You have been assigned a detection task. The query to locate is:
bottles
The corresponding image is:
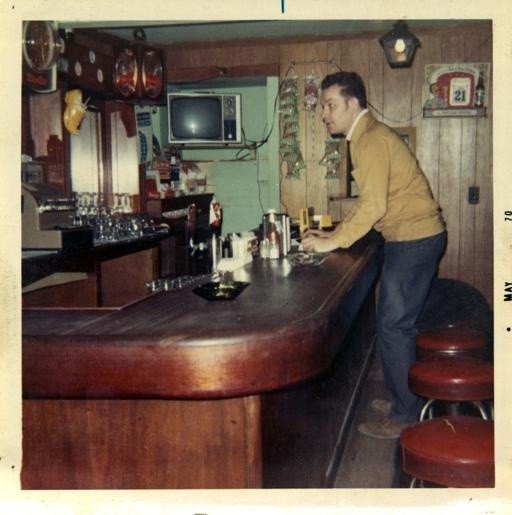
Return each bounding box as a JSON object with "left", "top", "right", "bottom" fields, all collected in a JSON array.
[
  {"left": 258, "top": 238, "right": 269, "bottom": 261},
  {"left": 474, "top": 70, "right": 485, "bottom": 108},
  {"left": 268, "top": 206, "right": 282, "bottom": 261}
]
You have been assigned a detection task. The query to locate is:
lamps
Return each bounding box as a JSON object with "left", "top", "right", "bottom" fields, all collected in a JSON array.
[{"left": 378, "top": 19, "right": 423, "bottom": 68}]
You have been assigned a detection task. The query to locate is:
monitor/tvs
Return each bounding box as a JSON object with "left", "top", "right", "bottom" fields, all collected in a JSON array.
[{"left": 167, "top": 93, "right": 243, "bottom": 143}]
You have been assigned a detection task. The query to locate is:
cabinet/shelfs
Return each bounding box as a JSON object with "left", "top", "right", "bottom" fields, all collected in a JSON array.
[{"left": 23, "top": 246, "right": 160, "bottom": 312}]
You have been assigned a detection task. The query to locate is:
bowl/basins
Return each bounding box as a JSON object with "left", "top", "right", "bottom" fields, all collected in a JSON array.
[{"left": 191, "top": 281, "right": 252, "bottom": 302}]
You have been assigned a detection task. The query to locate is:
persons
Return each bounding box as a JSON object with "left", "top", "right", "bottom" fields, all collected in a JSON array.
[
  {"left": 302, "top": 72, "right": 447, "bottom": 438},
  {"left": 424, "top": 83, "right": 448, "bottom": 108}
]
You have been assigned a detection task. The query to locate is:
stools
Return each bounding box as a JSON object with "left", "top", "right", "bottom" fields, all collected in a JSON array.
[{"left": 398, "top": 322, "right": 494, "bottom": 487}]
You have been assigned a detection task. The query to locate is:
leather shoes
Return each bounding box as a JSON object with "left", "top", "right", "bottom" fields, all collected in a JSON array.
[{"left": 356, "top": 398, "right": 401, "bottom": 440}]
